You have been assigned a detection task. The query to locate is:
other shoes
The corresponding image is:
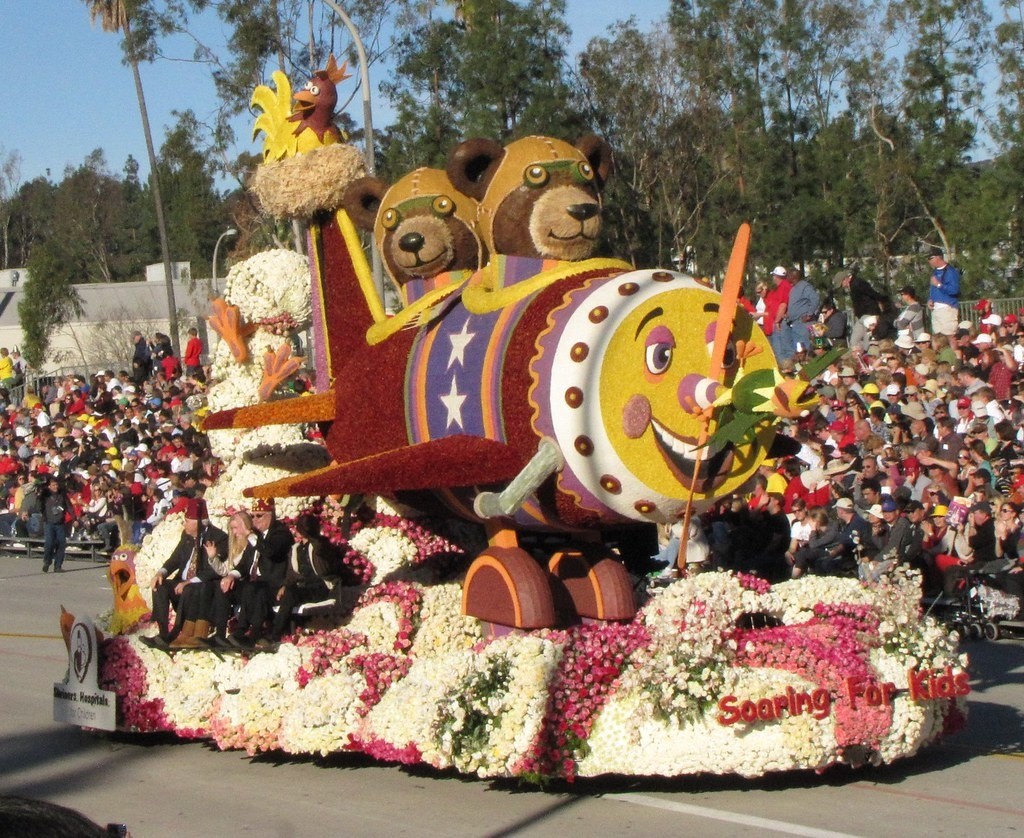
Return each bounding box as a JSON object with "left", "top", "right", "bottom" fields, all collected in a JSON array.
[
  {"left": 54, "top": 568, "right": 64, "bottom": 573},
  {"left": 42, "top": 563, "right": 49, "bottom": 572}
]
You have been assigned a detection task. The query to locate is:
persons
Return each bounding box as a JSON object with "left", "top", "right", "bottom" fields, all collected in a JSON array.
[
  {"left": 170, "top": 511, "right": 256, "bottom": 647},
  {"left": 230, "top": 513, "right": 342, "bottom": 655},
  {"left": 2, "top": 240, "right": 1024, "bottom": 658},
  {"left": 137, "top": 497, "right": 229, "bottom": 658},
  {"left": 194, "top": 497, "right": 296, "bottom": 653}
]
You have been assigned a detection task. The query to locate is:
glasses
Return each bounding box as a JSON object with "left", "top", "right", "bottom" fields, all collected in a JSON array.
[{"left": 249, "top": 512, "right": 267, "bottom": 519}]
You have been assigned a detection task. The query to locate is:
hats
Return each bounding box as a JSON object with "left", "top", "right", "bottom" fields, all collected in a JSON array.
[
  {"left": 762, "top": 246, "right": 1023, "bottom": 523},
  {"left": 76, "top": 414, "right": 89, "bottom": 421},
  {"left": 104, "top": 446, "right": 118, "bottom": 455},
  {"left": 184, "top": 499, "right": 208, "bottom": 519},
  {"left": 251, "top": 496, "right": 276, "bottom": 512},
  {"left": 5, "top": 404, "right": 16, "bottom": 410},
  {"left": 33, "top": 403, "right": 43, "bottom": 408},
  {"left": 147, "top": 397, "right": 161, "bottom": 405},
  {"left": 53, "top": 427, "right": 68, "bottom": 437},
  {"left": 101, "top": 460, "right": 110, "bottom": 464},
  {"left": 134, "top": 443, "right": 148, "bottom": 451},
  {"left": 95, "top": 370, "right": 105, "bottom": 377}
]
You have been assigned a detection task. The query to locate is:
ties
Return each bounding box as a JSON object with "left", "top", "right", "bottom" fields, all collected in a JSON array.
[
  {"left": 187, "top": 538, "right": 198, "bottom": 581},
  {"left": 301, "top": 545, "right": 307, "bottom": 577},
  {"left": 250, "top": 533, "right": 263, "bottom": 583}
]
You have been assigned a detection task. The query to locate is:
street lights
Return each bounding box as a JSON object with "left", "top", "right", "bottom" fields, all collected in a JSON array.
[{"left": 211, "top": 228, "right": 238, "bottom": 294}]
